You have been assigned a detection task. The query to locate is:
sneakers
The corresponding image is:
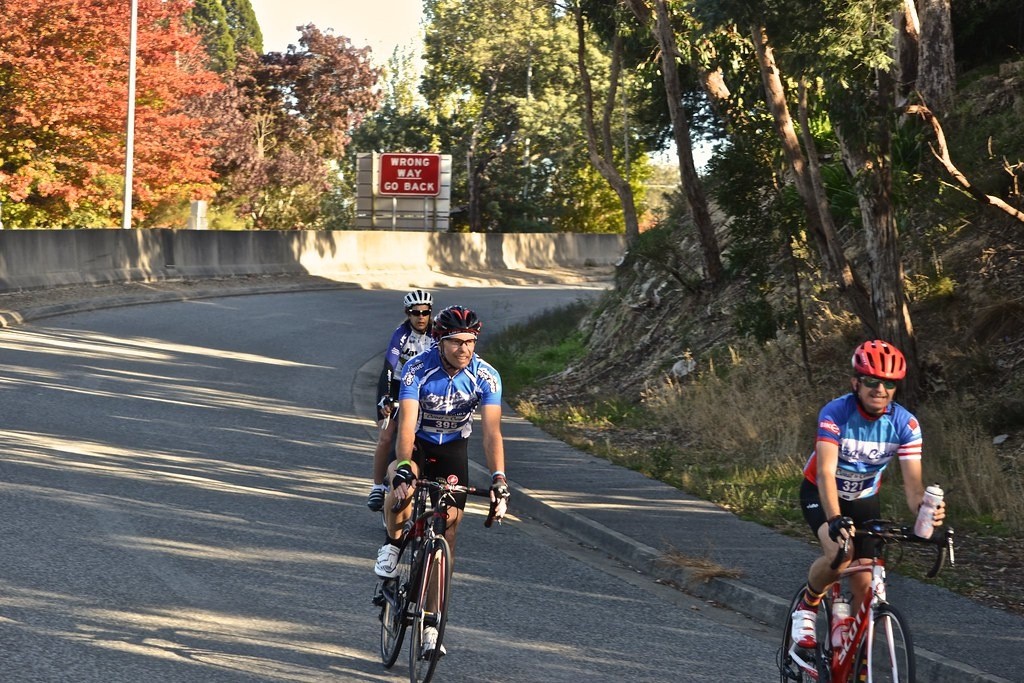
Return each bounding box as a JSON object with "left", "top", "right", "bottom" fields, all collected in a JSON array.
[
  {"left": 792, "top": 602, "right": 819, "bottom": 649},
  {"left": 374, "top": 544, "right": 401, "bottom": 579},
  {"left": 368, "top": 486, "right": 384, "bottom": 512},
  {"left": 421, "top": 627, "right": 446, "bottom": 661}
]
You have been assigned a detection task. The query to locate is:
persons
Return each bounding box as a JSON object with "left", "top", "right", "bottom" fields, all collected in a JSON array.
[
  {"left": 367, "top": 290, "right": 437, "bottom": 509},
  {"left": 374, "top": 305, "right": 509, "bottom": 662},
  {"left": 789, "top": 341, "right": 949, "bottom": 683}
]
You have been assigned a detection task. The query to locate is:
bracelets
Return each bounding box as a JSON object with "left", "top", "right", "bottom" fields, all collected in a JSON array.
[
  {"left": 492, "top": 472, "right": 506, "bottom": 480},
  {"left": 396, "top": 461, "right": 411, "bottom": 468}
]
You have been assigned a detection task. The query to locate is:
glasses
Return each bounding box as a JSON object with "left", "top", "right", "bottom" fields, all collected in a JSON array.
[
  {"left": 442, "top": 336, "right": 479, "bottom": 346},
  {"left": 409, "top": 309, "right": 432, "bottom": 316},
  {"left": 858, "top": 374, "right": 897, "bottom": 389}
]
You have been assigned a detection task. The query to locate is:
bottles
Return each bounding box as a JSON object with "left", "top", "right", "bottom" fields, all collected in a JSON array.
[
  {"left": 832, "top": 596, "right": 851, "bottom": 648},
  {"left": 915, "top": 482, "right": 944, "bottom": 539}
]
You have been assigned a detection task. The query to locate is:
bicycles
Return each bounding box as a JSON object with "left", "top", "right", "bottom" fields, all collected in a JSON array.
[
  {"left": 373, "top": 396, "right": 429, "bottom": 578},
  {"left": 774, "top": 516, "right": 956, "bottom": 683},
  {"left": 369, "top": 474, "right": 512, "bottom": 683}
]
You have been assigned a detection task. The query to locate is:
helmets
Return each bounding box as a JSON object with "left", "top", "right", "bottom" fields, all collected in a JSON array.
[
  {"left": 404, "top": 290, "right": 433, "bottom": 309},
  {"left": 432, "top": 305, "right": 483, "bottom": 344},
  {"left": 851, "top": 340, "right": 907, "bottom": 380}
]
